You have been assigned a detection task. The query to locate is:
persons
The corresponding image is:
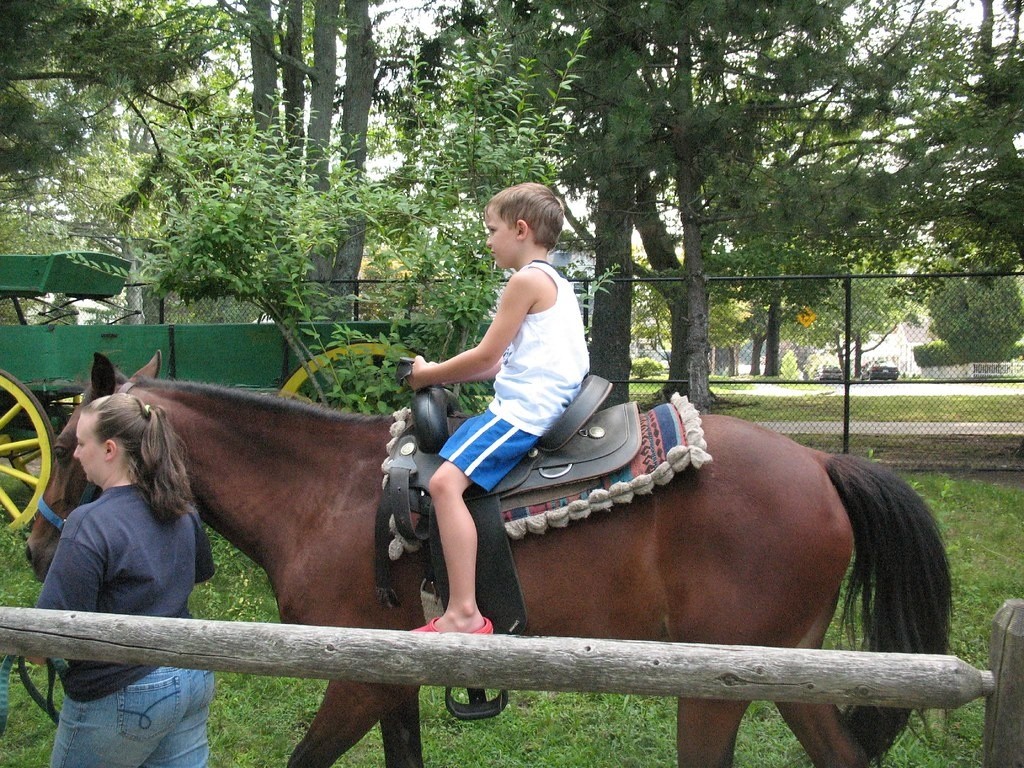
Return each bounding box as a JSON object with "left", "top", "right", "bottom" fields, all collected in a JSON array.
[
  {"left": 27, "top": 395, "right": 214, "bottom": 768},
  {"left": 405, "top": 182, "right": 592, "bottom": 633}
]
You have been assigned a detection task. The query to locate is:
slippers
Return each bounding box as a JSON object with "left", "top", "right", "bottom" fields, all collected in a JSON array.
[{"left": 412, "top": 618, "right": 493, "bottom": 634}]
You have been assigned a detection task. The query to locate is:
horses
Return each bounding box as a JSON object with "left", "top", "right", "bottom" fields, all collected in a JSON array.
[{"left": 24, "top": 351, "right": 950, "bottom": 768}]
